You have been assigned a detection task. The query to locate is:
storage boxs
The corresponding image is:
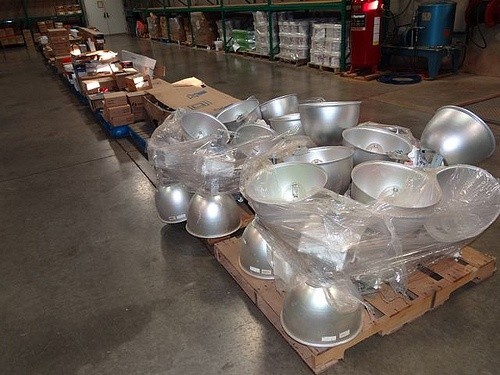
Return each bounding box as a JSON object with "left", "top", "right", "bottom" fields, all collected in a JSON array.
[{"left": 0, "top": 5, "right": 248, "bottom": 127}]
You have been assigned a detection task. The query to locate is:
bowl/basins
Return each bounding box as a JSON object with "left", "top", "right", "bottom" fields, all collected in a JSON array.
[
  {"left": 245, "top": 162, "right": 327, "bottom": 221},
  {"left": 419, "top": 105, "right": 495, "bottom": 166},
  {"left": 424, "top": 163, "right": 500, "bottom": 243},
  {"left": 340, "top": 125, "right": 412, "bottom": 162},
  {"left": 238, "top": 220, "right": 285, "bottom": 280},
  {"left": 187, "top": 190, "right": 243, "bottom": 241},
  {"left": 280, "top": 275, "right": 364, "bottom": 347},
  {"left": 215, "top": 99, "right": 261, "bottom": 131},
  {"left": 232, "top": 123, "right": 275, "bottom": 146},
  {"left": 258, "top": 92, "right": 302, "bottom": 120},
  {"left": 154, "top": 177, "right": 188, "bottom": 223},
  {"left": 279, "top": 146, "right": 354, "bottom": 197},
  {"left": 269, "top": 113, "right": 302, "bottom": 136},
  {"left": 299, "top": 99, "right": 363, "bottom": 145},
  {"left": 347, "top": 159, "right": 441, "bottom": 236},
  {"left": 178, "top": 113, "right": 229, "bottom": 150}
]
